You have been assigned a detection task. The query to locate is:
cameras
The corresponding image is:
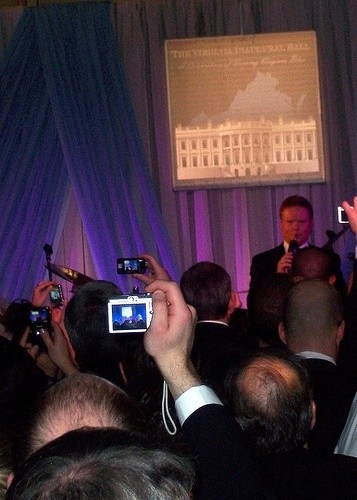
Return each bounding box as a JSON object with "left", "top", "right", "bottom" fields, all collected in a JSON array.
[
  {"left": 116, "top": 258, "right": 147, "bottom": 275},
  {"left": 106, "top": 293, "right": 153, "bottom": 334},
  {"left": 49, "top": 284, "right": 64, "bottom": 308},
  {"left": 337, "top": 206, "right": 354, "bottom": 224},
  {"left": 29, "top": 306, "right": 51, "bottom": 335}
]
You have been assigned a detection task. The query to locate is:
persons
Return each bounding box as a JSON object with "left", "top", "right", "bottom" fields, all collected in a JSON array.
[
  {"left": 8, "top": 280, "right": 257, "bottom": 500},
  {"left": 0, "top": 196, "right": 357, "bottom": 500},
  {"left": 247, "top": 195, "right": 349, "bottom": 331}
]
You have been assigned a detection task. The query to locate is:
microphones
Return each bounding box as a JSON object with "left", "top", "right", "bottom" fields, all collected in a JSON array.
[{"left": 287, "top": 239, "right": 298, "bottom": 273}]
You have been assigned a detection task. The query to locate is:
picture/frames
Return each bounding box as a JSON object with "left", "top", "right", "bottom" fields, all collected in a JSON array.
[{"left": 161, "top": 31, "right": 328, "bottom": 192}]
